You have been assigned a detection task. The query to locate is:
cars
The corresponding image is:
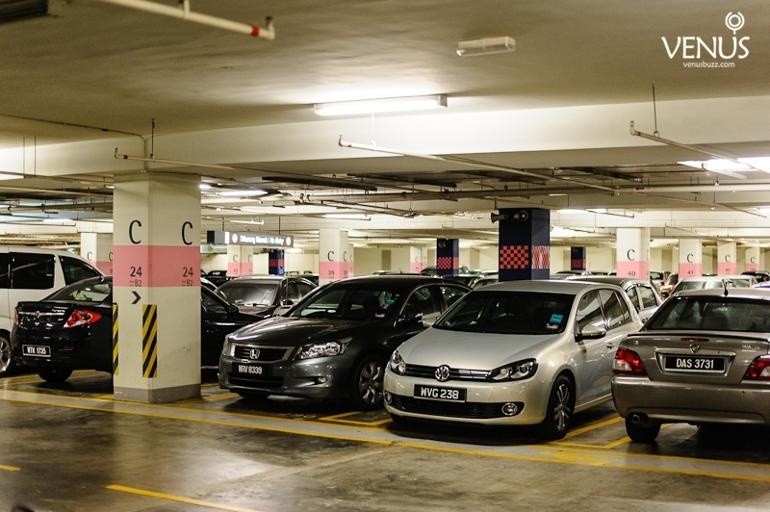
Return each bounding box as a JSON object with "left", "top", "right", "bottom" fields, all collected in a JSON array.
[{"left": 1, "top": 241, "right": 769, "bottom": 457}]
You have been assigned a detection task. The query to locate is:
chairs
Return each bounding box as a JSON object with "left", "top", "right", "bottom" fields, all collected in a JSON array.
[
  {"left": 362, "top": 296, "right": 380, "bottom": 317},
  {"left": 701, "top": 311, "right": 731, "bottom": 330}
]
